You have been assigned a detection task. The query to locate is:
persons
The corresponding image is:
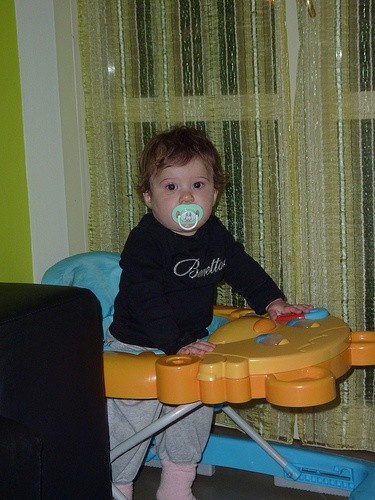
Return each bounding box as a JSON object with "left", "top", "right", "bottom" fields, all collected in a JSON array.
[{"left": 100, "top": 124, "right": 314, "bottom": 499}]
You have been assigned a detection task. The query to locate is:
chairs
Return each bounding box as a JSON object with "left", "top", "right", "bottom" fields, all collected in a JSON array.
[{"left": 38, "top": 253, "right": 226, "bottom": 415}]
